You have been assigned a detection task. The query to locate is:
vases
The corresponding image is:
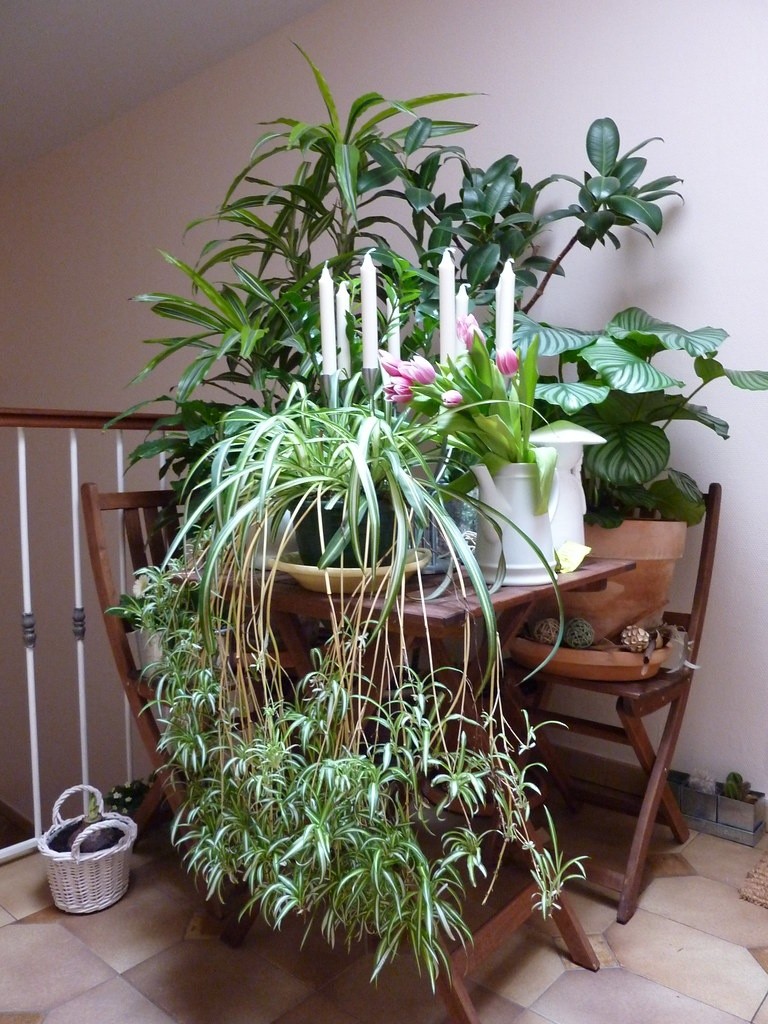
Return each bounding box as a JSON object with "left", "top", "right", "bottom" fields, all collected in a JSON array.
[{"left": 475, "top": 459, "right": 559, "bottom": 587}]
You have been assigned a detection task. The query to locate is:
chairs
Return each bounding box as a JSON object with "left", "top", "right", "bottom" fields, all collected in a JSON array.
[
  {"left": 82, "top": 480, "right": 350, "bottom": 920},
  {"left": 489, "top": 480, "right": 724, "bottom": 925}
]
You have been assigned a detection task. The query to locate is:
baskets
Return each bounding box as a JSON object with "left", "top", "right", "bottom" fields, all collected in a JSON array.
[{"left": 38, "top": 784, "right": 138, "bottom": 915}]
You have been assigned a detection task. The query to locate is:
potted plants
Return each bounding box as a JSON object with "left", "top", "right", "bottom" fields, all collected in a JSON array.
[{"left": 100, "top": 84, "right": 710, "bottom": 989}]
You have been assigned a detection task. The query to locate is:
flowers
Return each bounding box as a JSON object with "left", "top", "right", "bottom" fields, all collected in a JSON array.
[
  {"left": 379, "top": 311, "right": 544, "bottom": 464},
  {"left": 114, "top": 557, "right": 160, "bottom": 624}
]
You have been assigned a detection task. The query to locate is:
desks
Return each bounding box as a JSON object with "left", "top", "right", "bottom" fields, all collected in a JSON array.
[{"left": 168, "top": 558, "right": 639, "bottom": 1024}]
